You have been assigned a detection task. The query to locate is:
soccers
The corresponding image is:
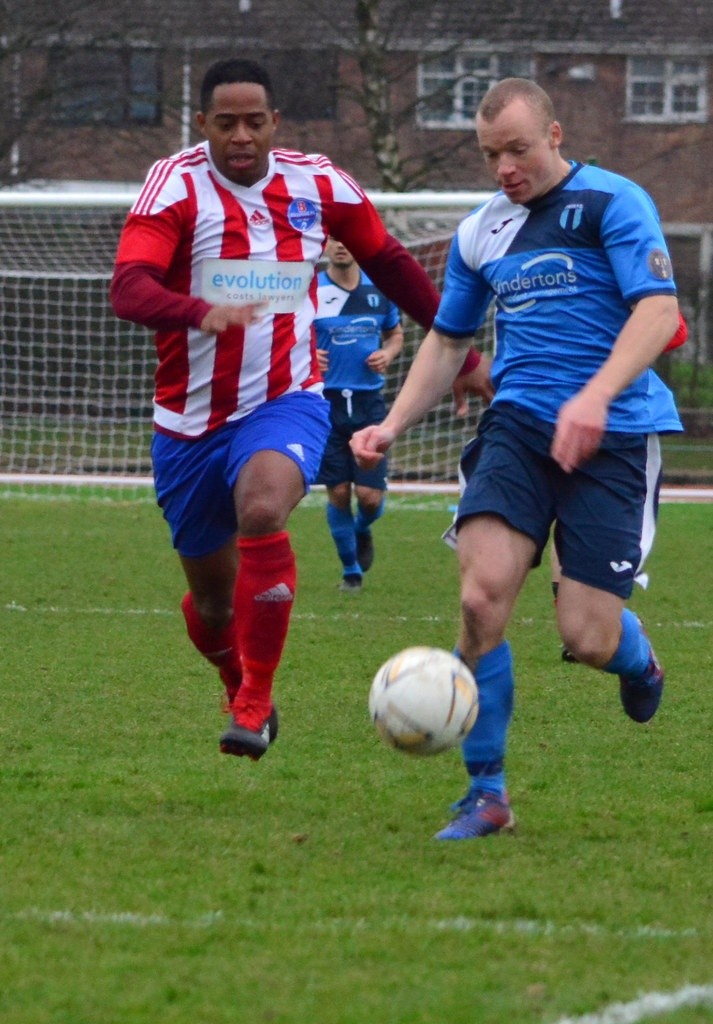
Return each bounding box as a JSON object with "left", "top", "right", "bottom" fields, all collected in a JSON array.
[{"left": 367, "top": 645, "right": 480, "bottom": 756}]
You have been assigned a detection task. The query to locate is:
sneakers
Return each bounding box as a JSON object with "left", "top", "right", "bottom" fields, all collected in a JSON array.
[
  {"left": 355, "top": 529, "right": 373, "bottom": 572},
  {"left": 219, "top": 707, "right": 278, "bottom": 760},
  {"left": 619, "top": 616, "right": 664, "bottom": 722},
  {"left": 430, "top": 786, "right": 518, "bottom": 839},
  {"left": 340, "top": 574, "right": 361, "bottom": 591}
]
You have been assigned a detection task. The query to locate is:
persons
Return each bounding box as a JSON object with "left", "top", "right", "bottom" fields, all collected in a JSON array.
[
  {"left": 346, "top": 78, "right": 688, "bottom": 847},
  {"left": 315, "top": 232, "right": 406, "bottom": 594},
  {"left": 108, "top": 59, "right": 497, "bottom": 761},
  {"left": 552, "top": 307, "right": 688, "bottom": 664}
]
